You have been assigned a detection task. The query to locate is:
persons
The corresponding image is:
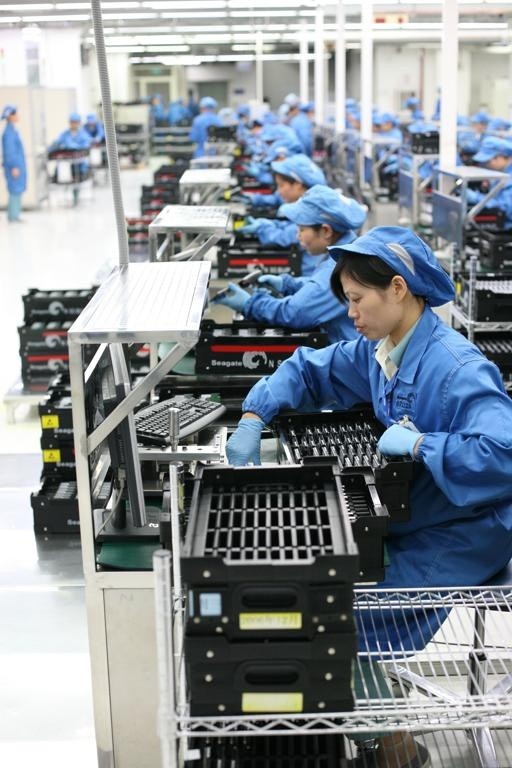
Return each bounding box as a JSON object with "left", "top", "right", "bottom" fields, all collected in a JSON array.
[
  {"left": 0, "top": 104, "right": 30, "bottom": 222},
  {"left": 47, "top": 79, "right": 511, "bottom": 344},
  {"left": 222, "top": 223, "right": 511, "bottom": 767}
]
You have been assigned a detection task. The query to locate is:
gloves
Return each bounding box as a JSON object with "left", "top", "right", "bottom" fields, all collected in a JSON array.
[
  {"left": 226, "top": 420, "right": 263, "bottom": 466},
  {"left": 242, "top": 163, "right": 272, "bottom": 237},
  {"left": 378, "top": 423, "right": 428, "bottom": 461},
  {"left": 260, "top": 273, "right": 284, "bottom": 294},
  {"left": 216, "top": 283, "right": 250, "bottom": 315}
]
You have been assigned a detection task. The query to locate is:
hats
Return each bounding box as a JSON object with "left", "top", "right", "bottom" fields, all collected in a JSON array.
[
  {"left": 346, "top": 93, "right": 511, "bottom": 161},
  {"left": 69, "top": 115, "right": 97, "bottom": 127},
  {"left": 269, "top": 153, "right": 325, "bottom": 188},
  {"left": 0, "top": 106, "right": 16, "bottom": 120},
  {"left": 327, "top": 225, "right": 456, "bottom": 306},
  {"left": 152, "top": 92, "right": 316, "bottom": 162},
  {"left": 276, "top": 185, "right": 369, "bottom": 232}
]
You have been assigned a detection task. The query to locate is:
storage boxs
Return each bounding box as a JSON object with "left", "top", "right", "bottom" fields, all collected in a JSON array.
[{"left": 17, "top": 286, "right": 113, "bottom": 539}]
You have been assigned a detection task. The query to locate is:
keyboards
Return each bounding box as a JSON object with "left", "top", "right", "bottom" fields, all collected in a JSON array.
[{"left": 133, "top": 393, "right": 227, "bottom": 448}]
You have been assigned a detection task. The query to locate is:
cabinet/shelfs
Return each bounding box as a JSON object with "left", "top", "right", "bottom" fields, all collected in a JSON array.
[{"left": 44, "top": 151, "right": 96, "bottom": 208}]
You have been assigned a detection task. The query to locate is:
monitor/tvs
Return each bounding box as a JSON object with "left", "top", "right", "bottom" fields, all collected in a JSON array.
[{"left": 94, "top": 341, "right": 162, "bottom": 543}]
